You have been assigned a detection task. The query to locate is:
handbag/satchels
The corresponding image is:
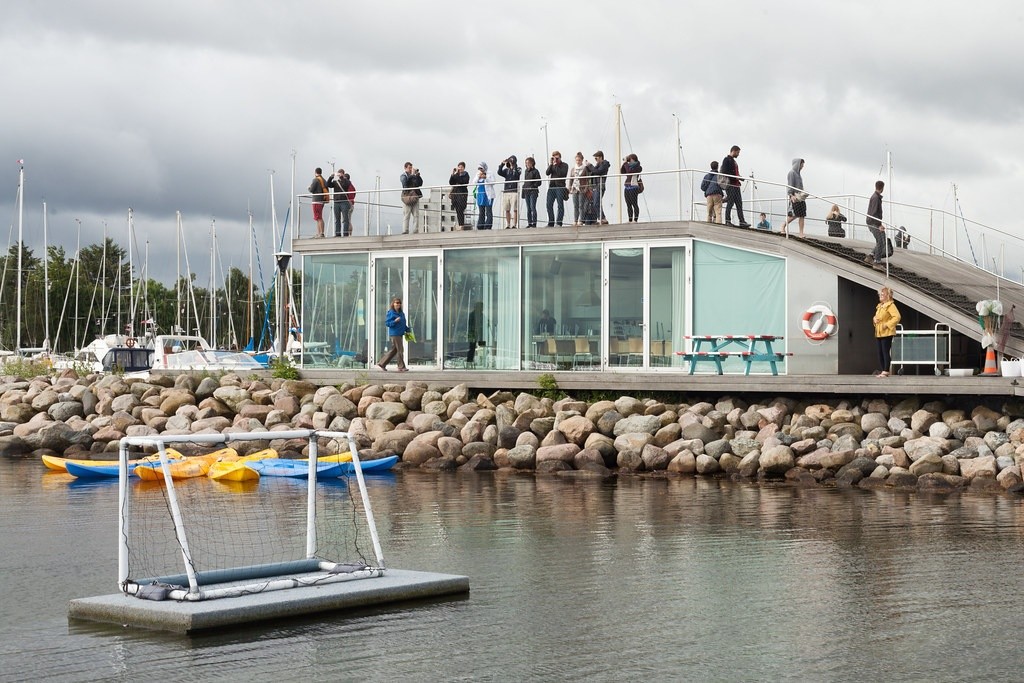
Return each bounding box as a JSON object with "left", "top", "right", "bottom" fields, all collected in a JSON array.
[
  {"left": 586, "top": 189, "right": 593, "bottom": 199},
  {"left": 449, "top": 191, "right": 453, "bottom": 199},
  {"left": 348, "top": 205, "right": 354, "bottom": 213},
  {"left": 881, "top": 238, "right": 893, "bottom": 258},
  {"left": 841, "top": 229, "right": 844, "bottom": 237},
  {"left": 564, "top": 189, "right": 569, "bottom": 201},
  {"left": 522, "top": 186, "right": 525, "bottom": 198},
  {"left": 637, "top": 179, "right": 644, "bottom": 194},
  {"left": 472, "top": 186, "right": 477, "bottom": 198},
  {"left": 317, "top": 177, "right": 330, "bottom": 202},
  {"left": 401, "top": 191, "right": 419, "bottom": 205},
  {"left": 716, "top": 175, "right": 728, "bottom": 189}
]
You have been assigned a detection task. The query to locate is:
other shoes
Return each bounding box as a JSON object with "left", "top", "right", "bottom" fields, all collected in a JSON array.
[
  {"left": 873, "top": 262, "right": 887, "bottom": 271},
  {"left": 876, "top": 372, "right": 889, "bottom": 377},
  {"left": 398, "top": 367, "right": 409, "bottom": 371},
  {"left": 595, "top": 220, "right": 609, "bottom": 224},
  {"left": 864, "top": 255, "right": 873, "bottom": 264},
  {"left": 740, "top": 222, "right": 751, "bottom": 227},
  {"left": 378, "top": 364, "right": 387, "bottom": 371},
  {"left": 727, "top": 222, "right": 734, "bottom": 227}
]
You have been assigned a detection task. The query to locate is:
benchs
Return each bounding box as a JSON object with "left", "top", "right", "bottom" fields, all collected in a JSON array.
[
  {"left": 774, "top": 352, "right": 794, "bottom": 362},
  {"left": 675, "top": 351, "right": 755, "bottom": 362}
]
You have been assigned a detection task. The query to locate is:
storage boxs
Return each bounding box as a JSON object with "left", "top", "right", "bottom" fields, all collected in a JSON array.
[{"left": 948, "top": 368, "right": 974, "bottom": 377}]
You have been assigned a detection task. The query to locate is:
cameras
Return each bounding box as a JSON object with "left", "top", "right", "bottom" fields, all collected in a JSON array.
[
  {"left": 623, "top": 156, "right": 630, "bottom": 161},
  {"left": 454, "top": 168, "right": 456, "bottom": 171},
  {"left": 416, "top": 169, "right": 419, "bottom": 172}
]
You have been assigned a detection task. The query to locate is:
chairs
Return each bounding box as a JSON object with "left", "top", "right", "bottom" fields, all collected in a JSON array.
[
  {"left": 539, "top": 337, "right": 672, "bottom": 371},
  {"left": 335, "top": 337, "right": 357, "bottom": 368},
  {"left": 362, "top": 339, "right": 486, "bottom": 366}
]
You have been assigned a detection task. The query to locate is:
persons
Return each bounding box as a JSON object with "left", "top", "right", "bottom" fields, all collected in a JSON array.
[
  {"left": 524, "top": 157, "right": 542, "bottom": 227},
  {"left": 497, "top": 155, "right": 522, "bottom": 229},
  {"left": 545, "top": 151, "right": 569, "bottom": 227},
  {"left": 308, "top": 167, "right": 356, "bottom": 237},
  {"left": 865, "top": 181, "right": 886, "bottom": 271},
  {"left": 720, "top": 146, "right": 751, "bottom": 228},
  {"left": 825, "top": 205, "right": 847, "bottom": 237},
  {"left": 895, "top": 225, "right": 910, "bottom": 249},
  {"left": 873, "top": 288, "right": 901, "bottom": 377},
  {"left": 568, "top": 151, "right": 610, "bottom": 226},
  {"left": 467, "top": 302, "right": 483, "bottom": 362},
  {"left": 781, "top": 158, "right": 806, "bottom": 238},
  {"left": 757, "top": 213, "right": 772, "bottom": 229},
  {"left": 448, "top": 162, "right": 471, "bottom": 229},
  {"left": 701, "top": 161, "right": 723, "bottom": 224},
  {"left": 378, "top": 298, "right": 408, "bottom": 372},
  {"left": 472, "top": 160, "right": 496, "bottom": 229},
  {"left": 400, "top": 162, "right": 423, "bottom": 234},
  {"left": 536, "top": 309, "right": 556, "bottom": 335},
  {"left": 621, "top": 154, "right": 642, "bottom": 222}
]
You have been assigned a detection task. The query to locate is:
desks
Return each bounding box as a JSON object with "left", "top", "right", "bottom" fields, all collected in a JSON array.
[{"left": 683, "top": 335, "right": 784, "bottom": 376}]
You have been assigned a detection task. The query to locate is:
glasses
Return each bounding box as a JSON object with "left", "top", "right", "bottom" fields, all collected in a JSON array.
[{"left": 394, "top": 302, "right": 401, "bottom": 304}]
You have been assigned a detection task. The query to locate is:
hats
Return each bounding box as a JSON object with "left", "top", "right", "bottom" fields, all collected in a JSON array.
[{"left": 593, "top": 151, "right": 603, "bottom": 157}]
